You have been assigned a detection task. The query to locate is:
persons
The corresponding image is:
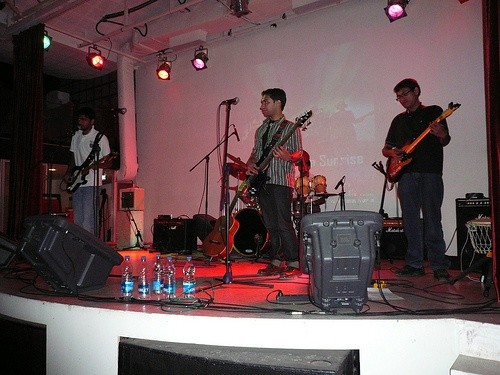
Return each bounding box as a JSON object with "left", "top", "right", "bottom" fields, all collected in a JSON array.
[
  {"left": 68, "top": 106, "right": 112, "bottom": 244},
  {"left": 382, "top": 75, "right": 453, "bottom": 282},
  {"left": 243, "top": 87, "right": 306, "bottom": 278}
]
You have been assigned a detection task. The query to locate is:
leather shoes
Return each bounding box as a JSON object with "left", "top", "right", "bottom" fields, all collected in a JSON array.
[
  {"left": 433, "top": 267, "right": 451, "bottom": 282},
  {"left": 257, "top": 264, "right": 281, "bottom": 276},
  {"left": 396, "top": 265, "right": 424, "bottom": 276},
  {"left": 280, "top": 266, "right": 302, "bottom": 278}
]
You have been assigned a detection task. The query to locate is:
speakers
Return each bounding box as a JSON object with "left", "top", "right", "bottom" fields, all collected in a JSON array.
[
  {"left": 455, "top": 198, "right": 493, "bottom": 269},
  {"left": 153, "top": 218, "right": 197, "bottom": 251},
  {"left": 18, "top": 216, "right": 125, "bottom": 294},
  {"left": 0, "top": 235, "right": 19, "bottom": 270},
  {"left": 298, "top": 210, "right": 384, "bottom": 309},
  {"left": 380, "top": 216, "right": 427, "bottom": 261}
]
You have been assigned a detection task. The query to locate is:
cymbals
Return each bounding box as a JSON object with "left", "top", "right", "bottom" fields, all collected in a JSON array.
[
  {"left": 226, "top": 153, "right": 248, "bottom": 168},
  {"left": 226, "top": 164, "right": 247, "bottom": 180}
]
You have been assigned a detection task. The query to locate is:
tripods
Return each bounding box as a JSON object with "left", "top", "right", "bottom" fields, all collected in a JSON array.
[{"left": 194, "top": 103, "right": 274, "bottom": 293}]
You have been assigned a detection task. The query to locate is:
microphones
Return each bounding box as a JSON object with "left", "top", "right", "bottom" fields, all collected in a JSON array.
[
  {"left": 111, "top": 108, "right": 126, "bottom": 114},
  {"left": 233, "top": 124, "right": 240, "bottom": 142},
  {"left": 221, "top": 97, "right": 239, "bottom": 105}
]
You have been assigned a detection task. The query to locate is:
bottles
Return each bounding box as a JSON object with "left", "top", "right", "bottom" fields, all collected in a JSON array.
[
  {"left": 137, "top": 256, "right": 151, "bottom": 300},
  {"left": 120, "top": 256, "right": 134, "bottom": 299},
  {"left": 151, "top": 254, "right": 165, "bottom": 294},
  {"left": 162, "top": 256, "right": 176, "bottom": 299},
  {"left": 183, "top": 257, "right": 196, "bottom": 298}
]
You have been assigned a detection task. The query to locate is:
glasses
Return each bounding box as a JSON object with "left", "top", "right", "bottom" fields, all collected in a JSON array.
[{"left": 396, "top": 89, "right": 413, "bottom": 102}]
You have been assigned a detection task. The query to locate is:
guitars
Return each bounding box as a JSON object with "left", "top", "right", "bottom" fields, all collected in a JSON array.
[
  {"left": 385, "top": 102, "right": 462, "bottom": 183},
  {"left": 66, "top": 150, "right": 118, "bottom": 194},
  {"left": 203, "top": 180, "right": 247, "bottom": 261},
  {"left": 244, "top": 109, "right": 314, "bottom": 196}
]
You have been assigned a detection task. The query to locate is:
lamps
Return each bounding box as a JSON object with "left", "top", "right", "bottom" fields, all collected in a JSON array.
[
  {"left": 191, "top": 45, "right": 209, "bottom": 71},
  {"left": 155, "top": 48, "right": 177, "bottom": 81},
  {"left": 43, "top": 30, "right": 53, "bottom": 52},
  {"left": 86, "top": 45, "right": 107, "bottom": 71},
  {"left": 230, "top": 0, "right": 252, "bottom": 18},
  {"left": 384, "top": 0, "right": 411, "bottom": 23}
]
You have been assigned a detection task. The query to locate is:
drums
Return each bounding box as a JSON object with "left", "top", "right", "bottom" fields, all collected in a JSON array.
[
  {"left": 233, "top": 208, "right": 281, "bottom": 258},
  {"left": 238, "top": 185, "right": 259, "bottom": 207},
  {"left": 294, "top": 177, "right": 311, "bottom": 194},
  {"left": 312, "top": 175, "right": 328, "bottom": 191}
]
